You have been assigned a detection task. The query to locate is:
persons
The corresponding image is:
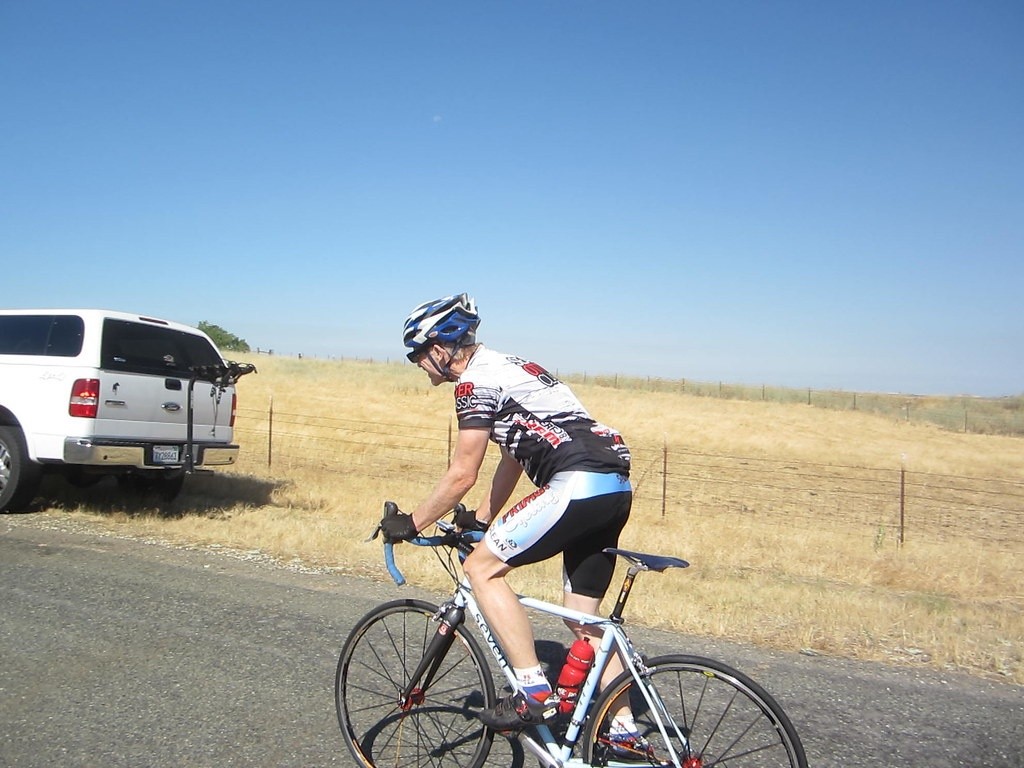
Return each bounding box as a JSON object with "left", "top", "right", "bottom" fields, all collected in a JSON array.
[{"left": 380, "top": 293, "right": 657, "bottom": 762}]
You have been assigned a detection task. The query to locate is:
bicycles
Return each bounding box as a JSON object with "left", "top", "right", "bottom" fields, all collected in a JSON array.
[{"left": 335, "top": 501, "right": 808, "bottom": 768}]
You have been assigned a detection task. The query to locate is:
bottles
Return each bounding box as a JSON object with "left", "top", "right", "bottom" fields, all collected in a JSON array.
[{"left": 554, "top": 638, "right": 594, "bottom": 713}]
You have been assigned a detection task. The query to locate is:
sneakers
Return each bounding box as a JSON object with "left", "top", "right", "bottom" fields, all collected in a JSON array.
[
  {"left": 573, "top": 731, "right": 653, "bottom": 763},
  {"left": 480, "top": 690, "right": 561, "bottom": 730}
]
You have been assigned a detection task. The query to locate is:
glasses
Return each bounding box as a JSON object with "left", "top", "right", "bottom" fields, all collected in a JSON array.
[{"left": 407, "top": 344, "right": 433, "bottom": 363}]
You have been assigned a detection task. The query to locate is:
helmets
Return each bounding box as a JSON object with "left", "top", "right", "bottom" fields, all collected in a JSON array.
[{"left": 403, "top": 293, "right": 481, "bottom": 347}]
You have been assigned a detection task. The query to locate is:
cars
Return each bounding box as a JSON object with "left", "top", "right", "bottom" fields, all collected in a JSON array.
[{"left": 0, "top": 309, "right": 257, "bottom": 514}]
